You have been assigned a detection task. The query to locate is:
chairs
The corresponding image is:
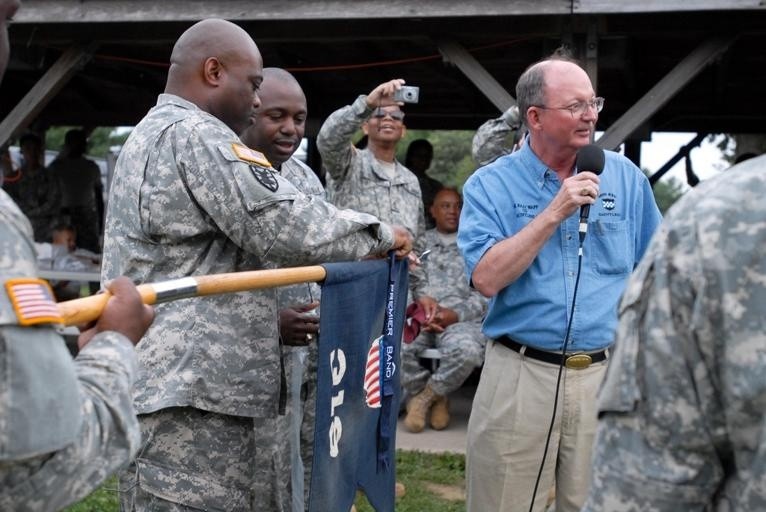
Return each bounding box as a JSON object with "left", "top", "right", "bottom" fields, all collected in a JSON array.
[{"left": 414, "top": 346, "right": 445, "bottom": 373}]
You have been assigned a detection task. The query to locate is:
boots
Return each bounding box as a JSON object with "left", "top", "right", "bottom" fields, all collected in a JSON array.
[
  {"left": 404, "top": 384, "right": 442, "bottom": 433},
  {"left": 431, "top": 397, "right": 449, "bottom": 430}
]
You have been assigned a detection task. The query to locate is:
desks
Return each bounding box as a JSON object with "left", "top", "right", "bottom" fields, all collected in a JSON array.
[{"left": 37, "top": 261, "right": 100, "bottom": 283}]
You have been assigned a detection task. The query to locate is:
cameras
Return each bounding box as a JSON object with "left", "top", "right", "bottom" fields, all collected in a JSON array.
[{"left": 394, "top": 86, "right": 419, "bottom": 104}]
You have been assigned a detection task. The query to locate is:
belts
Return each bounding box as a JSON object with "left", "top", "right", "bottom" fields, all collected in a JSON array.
[{"left": 498, "top": 336, "right": 614, "bottom": 370}]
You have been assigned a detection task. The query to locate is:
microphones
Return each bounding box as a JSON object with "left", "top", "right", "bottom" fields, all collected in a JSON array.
[{"left": 576, "top": 144, "right": 605, "bottom": 243}]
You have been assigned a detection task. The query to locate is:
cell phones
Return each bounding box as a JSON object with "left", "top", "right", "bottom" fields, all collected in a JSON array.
[{"left": 419, "top": 249, "right": 431, "bottom": 262}]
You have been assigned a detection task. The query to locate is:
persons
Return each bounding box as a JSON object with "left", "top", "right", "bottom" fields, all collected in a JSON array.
[
  {"left": 579, "top": 152, "right": 764, "bottom": 512},
  {"left": 1, "top": 17, "right": 666, "bottom": 511}
]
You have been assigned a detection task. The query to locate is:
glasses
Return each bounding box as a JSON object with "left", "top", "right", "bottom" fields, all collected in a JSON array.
[
  {"left": 366, "top": 109, "right": 405, "bottom": 120},
  {"left": 538, "top": 96, "right": 605, "bottom": 118}
]
